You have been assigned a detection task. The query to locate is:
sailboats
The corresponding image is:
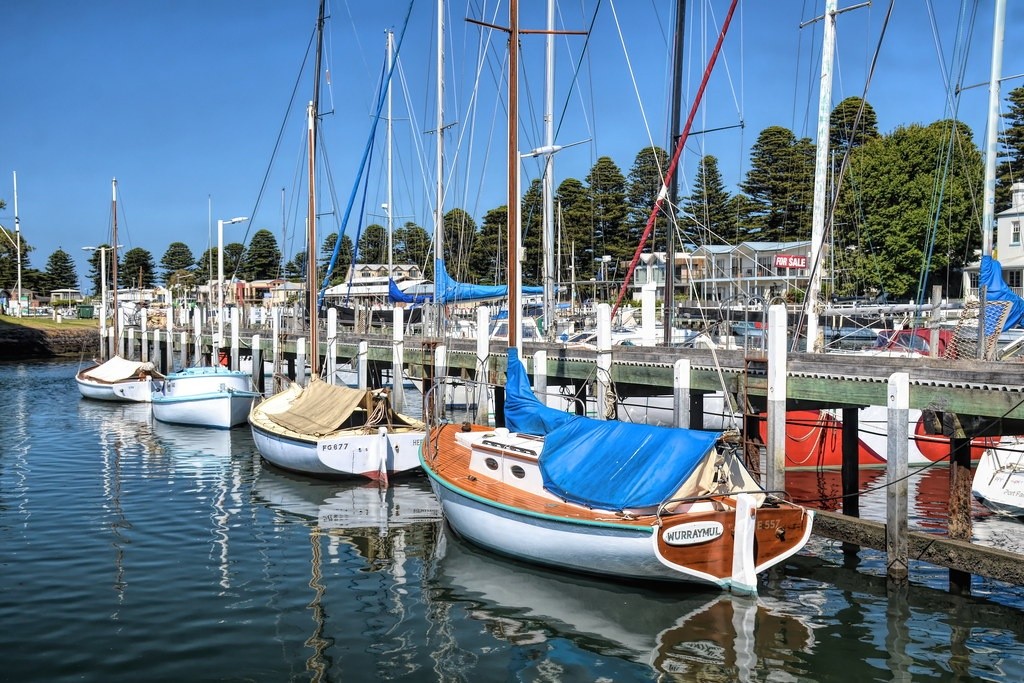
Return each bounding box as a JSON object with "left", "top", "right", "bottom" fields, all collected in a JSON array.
[{"left": 75, "top": 0, "right": 1024, "bottom": 585}]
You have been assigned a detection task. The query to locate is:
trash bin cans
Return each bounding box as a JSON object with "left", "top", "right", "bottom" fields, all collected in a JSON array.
[{"left": 76, "top": 305, "right": 94, "bottom": 320}]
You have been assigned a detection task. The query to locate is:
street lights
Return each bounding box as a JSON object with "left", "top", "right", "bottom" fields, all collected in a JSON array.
[
  {"left": 81, "top": 245, "right": 125, "bottom": 335},
  {"left": 217, "top": 216, "right": 250, "bottom": 349}
]
